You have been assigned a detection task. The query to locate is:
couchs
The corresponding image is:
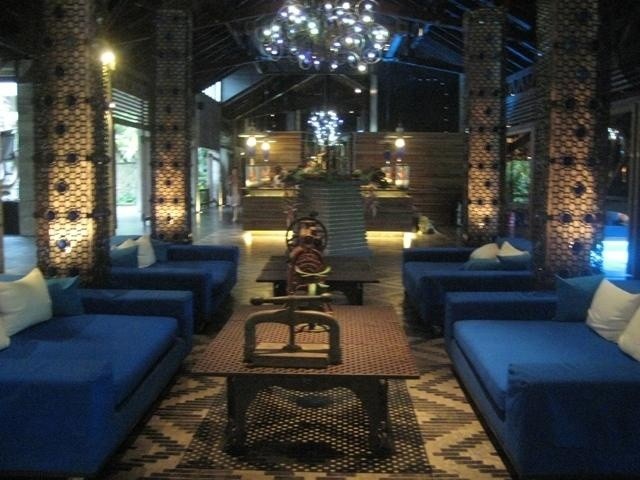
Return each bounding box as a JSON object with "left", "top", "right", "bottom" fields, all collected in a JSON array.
[
  {"left": 442, "top": 275, "right": 639, "bottom": 475},
  {"left": 402, "top": 234, "right": 535, "bottom": 332},
  {"left": 109, "top": 234, "right": 239, "bottom": 334},
  {"left": 0, "top": 283, "right": 196, "bottom": 480}
]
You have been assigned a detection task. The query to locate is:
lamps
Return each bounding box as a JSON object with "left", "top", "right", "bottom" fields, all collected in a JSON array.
[
  {"left": 255, "top": 0, "right": 394, "bottom": 75},
  {"left": 238, "top": 114, "right": 266, "bottom": 146},
  {"left": 257, "top": 137, "right": 278, "bottom": 150},
  {"left": 392, "top": 121, "right": 407, "bottom": 148},
  {"left": 306, "top": 79, "right": 343, "bottom": 147},
  {"left": 91, "top": 33, "right": 119, "bottom": 69}
]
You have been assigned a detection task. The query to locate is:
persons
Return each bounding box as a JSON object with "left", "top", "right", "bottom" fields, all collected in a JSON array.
[{"left": 224, "top": 166, "right": 243, "bottom": 223}]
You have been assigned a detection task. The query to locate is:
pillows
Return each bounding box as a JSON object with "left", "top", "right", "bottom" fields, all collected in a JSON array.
[
  {"left": 557, "top": 274, "right": 604, "bottom": 321},
  {"left": 460, "top": 241, "right": 530, "bottom": 272},
  {"left": 615, "top": 304, "right": 640, "bottom": 361},
  {"left": 45, "top": 274, "right": 80, "bottom": 315},
  {"left": 110, "top": 236, "right": 156, "bottom": 268},
  {"left": 111, "top": 245, "right": 139, "bottom": 268},
  {"left": 0, "top": 325, "right": 10, "bottom": 349},
  {"left": 0, "top": 266, "right": 54, "bottom": 336},
  {"left": 585, "top": 277, "right": 639, "bottom": 341}
]
